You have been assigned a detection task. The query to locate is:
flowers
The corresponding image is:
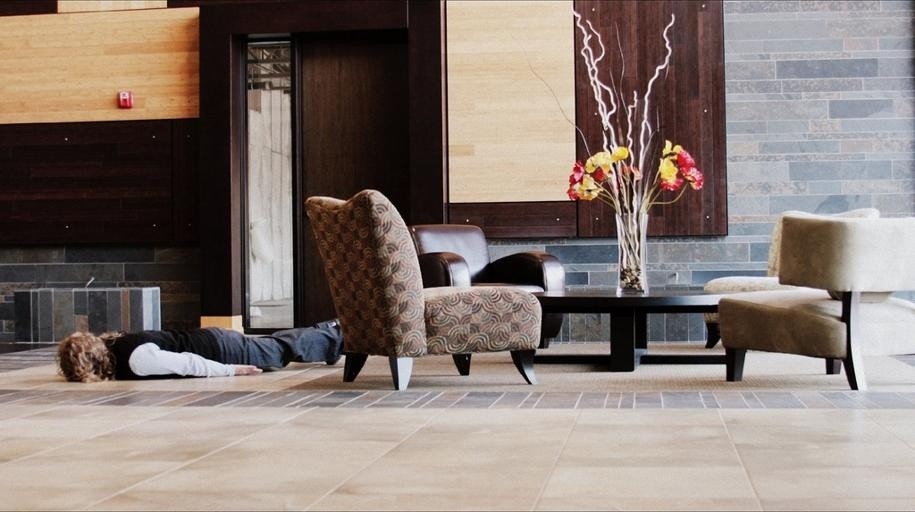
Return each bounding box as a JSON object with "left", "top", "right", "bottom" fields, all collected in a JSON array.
[{"left": 511, "top": 11, "right": 705, "bottom": 285}]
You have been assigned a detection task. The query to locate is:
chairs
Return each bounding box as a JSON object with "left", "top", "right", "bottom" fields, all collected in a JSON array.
[
  {"left": 408, "top": 224, "right": 566, "bottom": 348},
  {"left": 717, "top": 215, "right": 915, "bottom": 390},
  {"left": 703, "top": 208, "right": 880, "bottom": 350},
  {"left": 304, "top": 190, "right": 543, "bottom": 392}
]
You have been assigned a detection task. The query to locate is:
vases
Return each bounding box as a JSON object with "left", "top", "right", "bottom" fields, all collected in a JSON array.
[{"left": 616, "top": 213, "right": 649, "bottom": 297}]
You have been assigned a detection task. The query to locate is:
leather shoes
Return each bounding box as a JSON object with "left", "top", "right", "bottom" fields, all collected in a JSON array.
[{"left": 327, "top": 318, "right": 344, "bottom": 365}]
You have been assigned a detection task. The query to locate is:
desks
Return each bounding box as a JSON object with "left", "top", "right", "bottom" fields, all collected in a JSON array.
[{"left": 532, "top": 285, "right": 728, "bottom": 371}]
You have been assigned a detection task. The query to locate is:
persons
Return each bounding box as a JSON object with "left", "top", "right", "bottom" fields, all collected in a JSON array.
[{"left": 58, "top": 317, "right": 342, "bottom": 381}]
